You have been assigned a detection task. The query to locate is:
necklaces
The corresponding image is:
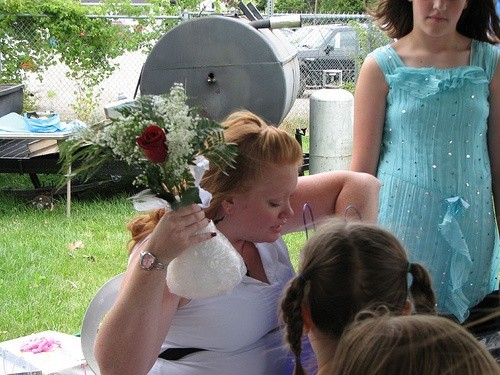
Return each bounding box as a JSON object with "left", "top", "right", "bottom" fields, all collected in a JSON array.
[{"left": 245, "top": 263, "right": 251, "bottom": 276}]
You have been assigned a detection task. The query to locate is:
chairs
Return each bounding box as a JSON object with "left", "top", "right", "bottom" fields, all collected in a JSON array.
[{"left": 79, "top": 269, "right": 129, "bottom": 375}]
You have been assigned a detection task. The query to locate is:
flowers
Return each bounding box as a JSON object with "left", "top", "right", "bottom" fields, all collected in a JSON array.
[{"left": 53, "top": 81, "right": 240, "bottom": 212}]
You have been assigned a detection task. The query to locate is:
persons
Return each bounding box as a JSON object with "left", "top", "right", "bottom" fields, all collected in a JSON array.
[
  {"left": 94, "top": 109, "right": 384, "bottom": 375},
  {"left": 281, "top": 218, "right": 438, "bottom": 375},
  {"left": 325, "top": 316, "right": 500, "bottom": 375},
  {"left": 354, "top": 0, "right": 499, "bottom": 321}
]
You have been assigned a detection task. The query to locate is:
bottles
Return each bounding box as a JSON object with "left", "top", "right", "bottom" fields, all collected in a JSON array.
[{"left": 32, "top": 197, "right": 49, "bottom": 211}]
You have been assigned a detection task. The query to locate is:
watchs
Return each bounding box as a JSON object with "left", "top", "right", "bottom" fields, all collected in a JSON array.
[{"left": 134, "top": 245, "right": 168, "bottom": 273}]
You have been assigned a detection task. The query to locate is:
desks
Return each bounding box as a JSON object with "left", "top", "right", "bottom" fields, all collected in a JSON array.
[
  {"left": 0, "top": 123, "right": 104, "bottom": 217},
  {"left": 0, "top": 330, "right": 96, "bottom": 375}
]
